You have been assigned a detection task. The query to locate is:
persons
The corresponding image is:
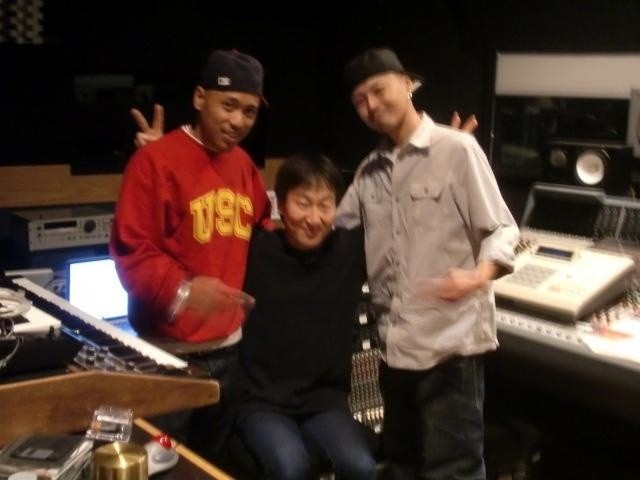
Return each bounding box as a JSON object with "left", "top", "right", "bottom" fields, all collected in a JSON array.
[
  {"left": 330, "top": 46, "right": 524, "bottom": 480},
  {"left": 107, "top": 47, "right": 279, "bottom": 475},
  {"left": 124, "top": 101, "right": 480, "bottom": 480}
]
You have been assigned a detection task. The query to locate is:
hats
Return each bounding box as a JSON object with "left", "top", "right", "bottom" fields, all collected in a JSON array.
[
  {"left": 341, "top": 46, "right": 426, "bottom": 95},
  {"left": 199, "top": 47, "right": 271, "bottom": 109}
]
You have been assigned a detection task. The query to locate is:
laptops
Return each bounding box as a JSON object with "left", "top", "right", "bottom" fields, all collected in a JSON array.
[{"left": 65, "top": 254, "right": 140, "bottom": 346}]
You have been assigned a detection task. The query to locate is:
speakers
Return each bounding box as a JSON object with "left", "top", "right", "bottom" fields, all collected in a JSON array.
[{"left": 536, "top": 139, "right": 635, "bottom": 194}]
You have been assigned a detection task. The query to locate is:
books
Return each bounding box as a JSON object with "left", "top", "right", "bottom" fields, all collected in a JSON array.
[{"left": 1, "top": 428, "right": 95, "bottom": 480}]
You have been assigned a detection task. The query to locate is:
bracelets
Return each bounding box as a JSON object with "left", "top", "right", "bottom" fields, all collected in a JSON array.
[{"left": 164, "top": 280, "right": 195, "bottom": 327}]
